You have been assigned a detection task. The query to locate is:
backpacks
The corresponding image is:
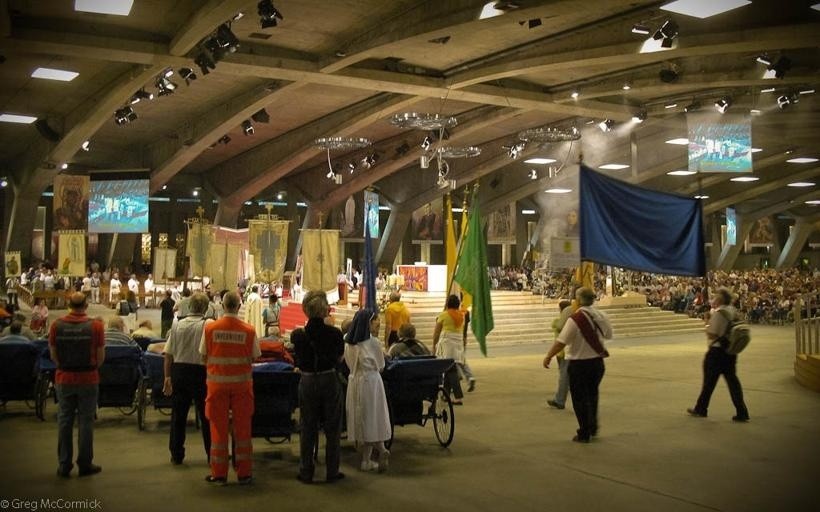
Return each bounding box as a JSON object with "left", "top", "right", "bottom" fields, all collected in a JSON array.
[{"left": 714, "top": 309, "right": 751, "bottom": 359}]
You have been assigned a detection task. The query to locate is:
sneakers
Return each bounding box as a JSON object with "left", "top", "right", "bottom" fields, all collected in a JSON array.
[
  {"left": 572, "top": 429, "right": 596, "bottom": 442},
  {"left": 378, "top": 449, "right": 390, "bottom": 473},
  {"left": 207, "top": 474, "right": 226, "bottom": 486},
  {"left": 361, "top": 460, "right": 379, "bottom": 470},
  {"left": 441, "top": 377, "right": 475, "bottom": 405},
  {"left": 548, "top": 401, "right": 565, "bottom": 409},
  {"left": 732, "top": 416, "right": 749, "bottom": 421},
  {"left": 296, "top": 475, "right": 313, "bottom": 483},
  {"left": 325, "top": 473, "right": 345, "bottom": 483},
  {"left": 237, "top": 474, "right": 253, "bottom": 484},
  {"left": 78, "top": 466, "right": 101, "bottom": 477},
  {"left": 686, "top": 408, "right": 706, "bottom": 417}
]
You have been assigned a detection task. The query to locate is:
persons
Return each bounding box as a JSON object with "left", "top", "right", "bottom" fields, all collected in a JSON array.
[
  {"left": 196, "top": 291, "right": 262, "bottom": 488},
  {"left": 545, "top": 297, "right": 580, "bottom": 412},
  {"left": 48, "top": 291, "right": 105, "bottom": 480},
  {"left": 485, "top": 260, "right": 820, "bottom": 328},
  {"left": 290, "top": 289, "right": 348, "bottom": 485},
  {"left": 0, "top": 253, "right": 476, "bottom": 407},
  {"left": 691, "top": 134, "right": 736, "bottom": 161},
  {"left": 161, "top": 295, "right": 219, "bottom": 468},
  {"left": 103, "top": 194, "right": 138, "bottom": 221},
  {"left": 343, "top": 309, "right": 397, "bottom": 472},
  {"left": 685, "top": 286, "right": 753, "bottom": 424},
  {"left": 542, "top": 286, "right": 613, "bottom": 443}
]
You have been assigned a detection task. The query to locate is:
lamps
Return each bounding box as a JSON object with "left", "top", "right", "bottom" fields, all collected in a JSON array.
[{"left": 113, "top": 0, "right": 283, "bottom": 143}]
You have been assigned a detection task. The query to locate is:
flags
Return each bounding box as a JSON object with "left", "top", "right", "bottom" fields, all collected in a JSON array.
[
  {"left": 444, "top": 178, "right": 496, "bottom": 358},
  {"left": 358, "top": 197, "right": 379, "bottom": 316},
  {"left": 441, "top": 186, "right": 460, "bottom": 317},
  {"left": 458, "top": 184, "right": 474, "bottom": 315},
  {"left": 576, "top": 163, "right": 708, "bottom": 279}
]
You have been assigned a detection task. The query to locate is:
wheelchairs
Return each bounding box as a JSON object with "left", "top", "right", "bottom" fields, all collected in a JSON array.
[
  {"left": 1, "top": 335, "right": 455, "bottom": 447},
  {"left": 655, "top": 299, "right": 819, "bottom": 328}
]
